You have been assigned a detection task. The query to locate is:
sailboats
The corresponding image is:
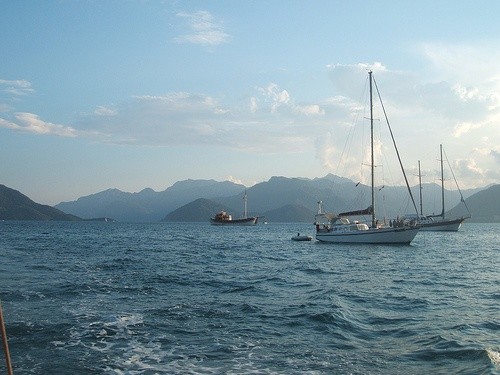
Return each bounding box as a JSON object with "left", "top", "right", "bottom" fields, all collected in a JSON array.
[
  {"left": 312, "top": 71, "right": 422, "bottom": 247},
  {"left": 403, "top": 143, "right": 471, "bottom": 233}
]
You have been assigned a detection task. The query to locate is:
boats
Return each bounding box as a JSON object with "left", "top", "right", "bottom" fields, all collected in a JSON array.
[
  {"left": 263, "top": 221, "right": 268, "bottom": 224},
  {"left": 291, "top": 235, "right": 313, "bottom": 241},
  {"left": 207, "top": 188, "right": 258, "bottom": 225}
]
